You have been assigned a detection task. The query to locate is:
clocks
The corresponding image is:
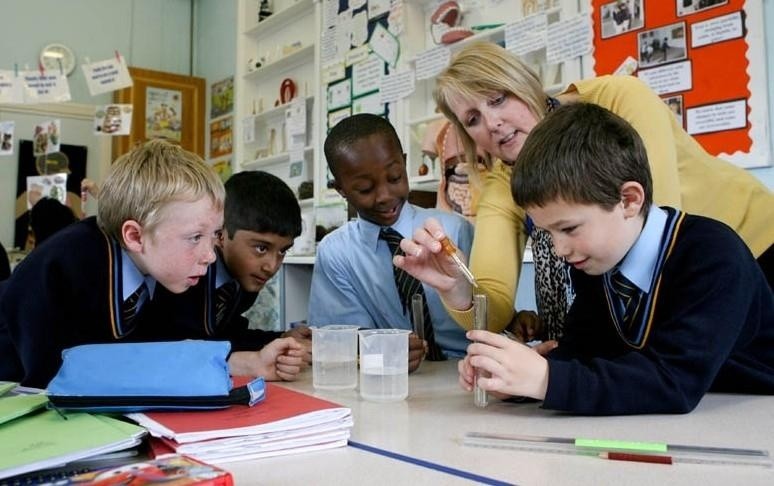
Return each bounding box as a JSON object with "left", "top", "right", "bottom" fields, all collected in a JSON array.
[{"left": 39, "top": 43, "right": 76, "bottom": 77}]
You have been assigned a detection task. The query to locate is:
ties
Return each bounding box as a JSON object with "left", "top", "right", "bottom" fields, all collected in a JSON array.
[
  {"left": 609, "top": 270, "right": 645, "bottom": 333},
  {"left": 214, "top": 281, "right": 237, "bottom": 324},
  {"left": 120, "top": 280, "right": 146, "bottom": 335},
  {"left": 379, "top": 229, "right": 450, "bottom": 361}
]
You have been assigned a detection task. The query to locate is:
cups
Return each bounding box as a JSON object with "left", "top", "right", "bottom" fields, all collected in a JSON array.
[{"left": 310, "top": 323, "right": 413, "bottom": 401}]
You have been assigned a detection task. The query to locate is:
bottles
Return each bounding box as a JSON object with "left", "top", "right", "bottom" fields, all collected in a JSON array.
[
  {"left": 412, "top": 293, "right": 424, "bottom": 341},
  {"left": 473, "top": 294, "right": 489, "bottom": 408}
]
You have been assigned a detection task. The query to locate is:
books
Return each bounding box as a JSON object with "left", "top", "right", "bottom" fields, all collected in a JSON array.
[{"left": 0, "top": 373, "right": 357, "bottom": 486}]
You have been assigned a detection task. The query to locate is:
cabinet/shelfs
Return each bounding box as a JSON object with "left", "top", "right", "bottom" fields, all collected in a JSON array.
[{"left": 230, "top": 0, "right": 329, "bottom": 267}]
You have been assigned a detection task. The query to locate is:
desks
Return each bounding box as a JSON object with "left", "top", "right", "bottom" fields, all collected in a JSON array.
[{"left": 198, "top": 356, "right": 774, "bottom": 481}]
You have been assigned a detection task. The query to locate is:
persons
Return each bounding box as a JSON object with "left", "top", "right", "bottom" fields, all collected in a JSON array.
[
  {"left": 612, "top": 0, "right": 633, "bottom": 33},
  {"left": 308, "top": 112, "right": 474, "bottom": 377},
  {"left": 0, "top": 139, "right": 306, "bottom": 392},
  {"left": 455, "top": 100, "right": 773, "bottom": 417},
  {"left": 391, "top": 39, "right": 774, "bottom": 335},
  {"left": 27, "top": 189, "right": 78, "bottom": 249},
  {"left": 182, "top": 169, "right": 320, "bottom": 374},
  {"left": 641, "top": 36, "right": 670, "bottom": 63},
  {"left": 80, "top": 178, "right": 99, "bottom": 200}
]
